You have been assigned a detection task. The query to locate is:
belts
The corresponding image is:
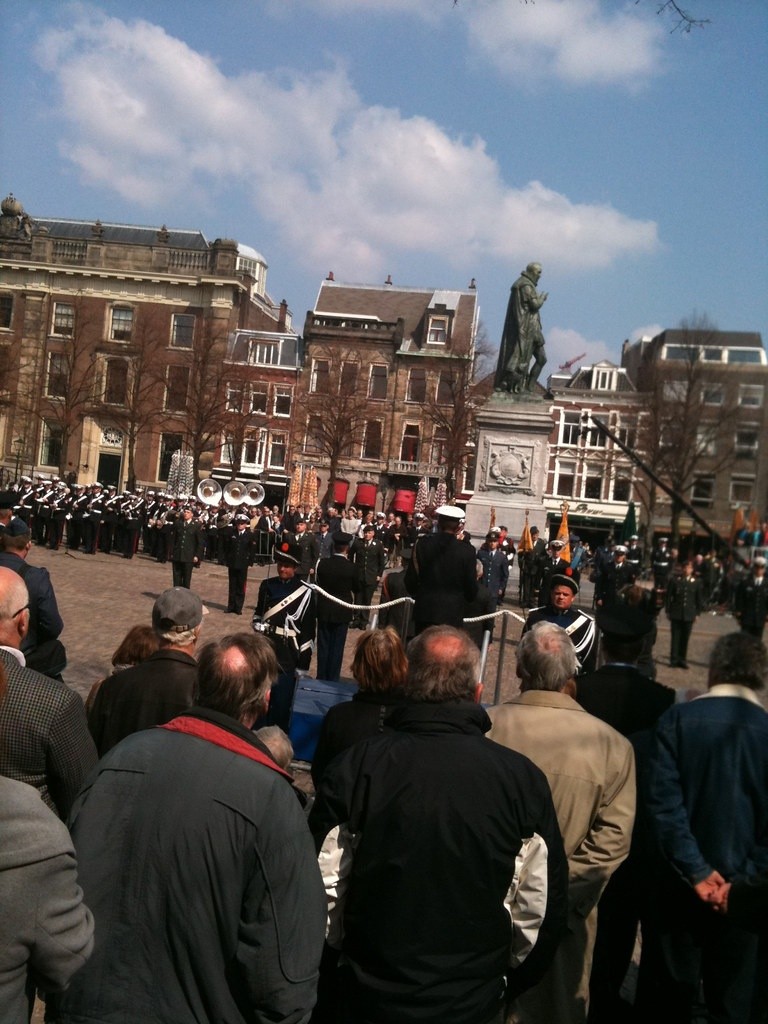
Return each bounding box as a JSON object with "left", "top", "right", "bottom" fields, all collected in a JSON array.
[{"left": 261, "top": 622, "right": 297, "bottom": 638}]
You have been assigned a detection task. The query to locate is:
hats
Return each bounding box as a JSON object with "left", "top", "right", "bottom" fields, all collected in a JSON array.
[
  {"left": 19, "top": 474, "right": 220, "bottom": 507},
  {"left": 184, "top": 506, "right": 193, "bottom": 512},
  {"left": 490, "top": 527, "right": 501, "bottom": 532},
  {"left": 332, "top": 531, "right": 353, "bottom": 543},
  {"left": 414, "top": 514, "right": 426, "bottom": 520},
  {"left": 611, "top": 545, "right": 628, "bottom": 556},
  {"left": 434, "top": 506, "right": 465, "bottom": 521},
  {"left": 363, "top": 525, "right": 374, "bottom": 531},
  {"left": 4, "top": 517, "right": 28, "bottom": 537},
  {"left": 376, "top": 512, "right": 386, "bottom": 518},
  {"left": 658, "top": 538, "right": 669, "bottom": 543},
  {"left": 754, "top": 556, "right": 767, "bottom": 567},
  {"left": 153, "top": 586, "right": 210, "bottom": 632},
  {"left": 486, "top": 532, "right": 499, "bottom": 541},
  {"left": 236, "top": 514, "right": 250, "bottom": 523},
  {"left": 550, "top": 540, "right": 566, "bottom": 551},
  {"left": 629, "top": 535, "right": 639, "bottom": 541},
  {"left": 275, "top": 543, "right": 303, "bottom": 566},
  {"left": 549, "top": 575, "right": 579, "bottom": 595},
  {"left": 296, "top": 518, "right": 306, "bottom": 524},
  {"left": 320, "top": 519, "right": 330, "bottom": 527}
]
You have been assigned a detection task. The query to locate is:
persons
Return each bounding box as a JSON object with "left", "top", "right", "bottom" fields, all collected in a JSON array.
[
  {"left": 575, "top": 596, "right": 675, "bottom": 1024},
  {"left": 639, "top": 631, "right": 768, "bottom": 1024},
  {"left": 0, "top": 470, "right": 768, "bottom": 736},
  {"left": 492, "top": 263, "right": 548, "bottom": 392},
  {"left": 485, "top": 619, "right": 636, "bottom": 1024},
  {"left": 0, "top": 508, "right": 98, "bottom": 1024},
  {"left": 306, "top": 626, "right": 569, "bottom": 1024},
  {"left": 42, "top": 586, "right": 327, "bottom": 1024}
]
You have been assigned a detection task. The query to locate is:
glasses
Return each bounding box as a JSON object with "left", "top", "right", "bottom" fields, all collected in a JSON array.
[
  {"left": 237, "top": 522, "right": 244, "bottom": 525},
  {"left": 11, "top": 599, "right": 31, "bottom": 619}
]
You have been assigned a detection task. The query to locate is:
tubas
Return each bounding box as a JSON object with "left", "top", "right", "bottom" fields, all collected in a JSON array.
[{"left": 197, "top": 476, "right": 266, "bottom": 529}]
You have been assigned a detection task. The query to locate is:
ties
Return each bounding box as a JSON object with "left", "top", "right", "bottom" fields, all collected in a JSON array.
[
  {"left": 185, "top": 522, "right": 188, "bottom": 526},
  {"left": 298, "top": 534, "right": 301, "bottom": 539},
  {"left": 554, "top": 561, "right": 557, "bottom": 567},
  {"left": 322, "top": 535, "right": 324, "bottom": 539},
  {"left": 617, "top": 565, "right": 619, "bottom": 569}
]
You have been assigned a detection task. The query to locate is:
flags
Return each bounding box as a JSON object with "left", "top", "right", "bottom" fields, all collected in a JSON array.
[{"left": 556, "top": 510, "right": 571, "bottom": 563}]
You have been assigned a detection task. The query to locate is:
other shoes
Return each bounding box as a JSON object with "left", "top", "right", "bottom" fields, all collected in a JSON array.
[
  {"left": 350, "top": 623, "right": 366, "bottom": 631},
  {"left": 224, "top": 608, "right": 242, "bottom": 615},
  {"left": 668, "top": 662, "right": 689, "bottom": 670}
]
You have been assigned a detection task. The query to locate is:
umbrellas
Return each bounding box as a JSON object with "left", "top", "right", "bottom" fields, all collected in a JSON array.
[
  {"left": 432, "top": 478, "right": 447, "bottom": 509},
  {"left": 166, "top": 449, "right": 194, "bottom": 496},
  {"left": 286, "top": 460, "right": 318, "bottom": 508},
  {"left": 414, "top": 475, "right": 429, "bottom": 517},
  {"left": 618, "top": 502, "right": 636, "bottom": 545}
]
